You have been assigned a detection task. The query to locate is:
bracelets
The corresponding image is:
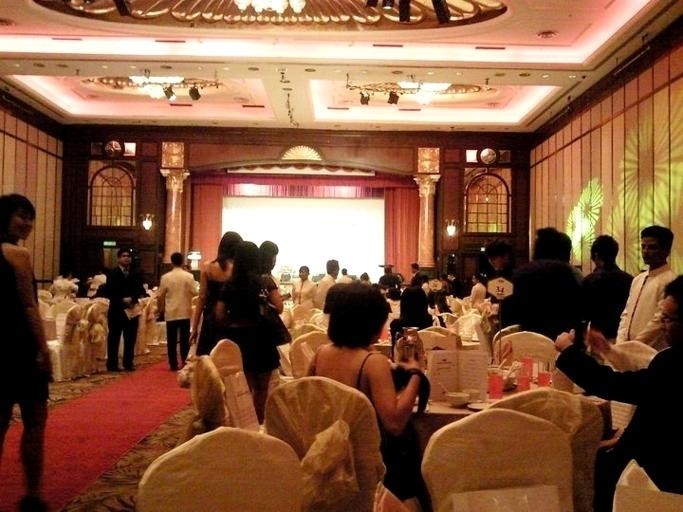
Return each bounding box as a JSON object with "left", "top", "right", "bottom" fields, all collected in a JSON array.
[{"left": 40, "top": 347, "right": 49, "bottom": 357}]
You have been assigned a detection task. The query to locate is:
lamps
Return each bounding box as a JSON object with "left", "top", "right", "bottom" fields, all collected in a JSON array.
[
  {"left": 365, "top": 0, "right": 453, "bottom": 27},
  {"left": 165, "top": 80, "right": 203, "bottom": 104},
  {"left": 357, "top": 87, "right": 402, "bottom": 107}
]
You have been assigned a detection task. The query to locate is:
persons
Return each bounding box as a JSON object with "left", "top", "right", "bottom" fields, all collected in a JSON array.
[
  {"left": 1, "top": 193, "right": 55, "bottom": 512},
  {"left": 157, "top": 252, "right": 199, "bottom": 369},
  {"left": 291, "top": 260, "right": 470, "bottom": 512},
  {"left": 107, "top": 247, "right": 146, "bottom": 370},
  {"left": 188, "top": 231, "right": 292, "bottom": 423},
  {"left": 469, "top": 225, "right": 683, "bottom": 511}
]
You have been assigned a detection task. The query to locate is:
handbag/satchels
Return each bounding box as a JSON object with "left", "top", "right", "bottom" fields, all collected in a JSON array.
[{"left": 257, "top": 293, "right": 292, "bottom": 345}]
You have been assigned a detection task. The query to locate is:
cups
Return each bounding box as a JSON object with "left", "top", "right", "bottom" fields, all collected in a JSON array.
[
  {"left": 538, "top": 360, "right": 551, "bottom": 387},
  {"left": 520, "top": 352, "right": 533, "bottom": 382},
  {"left": 516, "top": 365, "right": 530, "bottom": 391},
  {"left": 487, "top": 368, "right": 503, "bottom": 399},
  {"left": 462, "top": 389, "right": 480, "bottom": 401}
]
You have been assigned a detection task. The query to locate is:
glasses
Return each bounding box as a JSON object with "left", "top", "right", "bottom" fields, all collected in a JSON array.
[{"left": 661, "top": 315, "right": 679, "bottom": 323}]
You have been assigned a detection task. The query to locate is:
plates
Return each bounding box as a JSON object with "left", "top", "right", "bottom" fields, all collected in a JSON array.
[{"left": 466, "top": 403, "right": 487, "bottom": 410}]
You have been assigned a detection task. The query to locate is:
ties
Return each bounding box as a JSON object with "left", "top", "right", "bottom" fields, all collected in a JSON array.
[{"left": 124, "top": 267, "right": 127, "bottom": 277}]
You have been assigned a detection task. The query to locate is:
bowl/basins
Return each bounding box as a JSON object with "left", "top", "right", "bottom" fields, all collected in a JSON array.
[
  {"left": 444, "top": 391, "right": 469, "bottom": 407},
  {"left": 502, "top": 370, "right": 515, "bottom": 390}
]
